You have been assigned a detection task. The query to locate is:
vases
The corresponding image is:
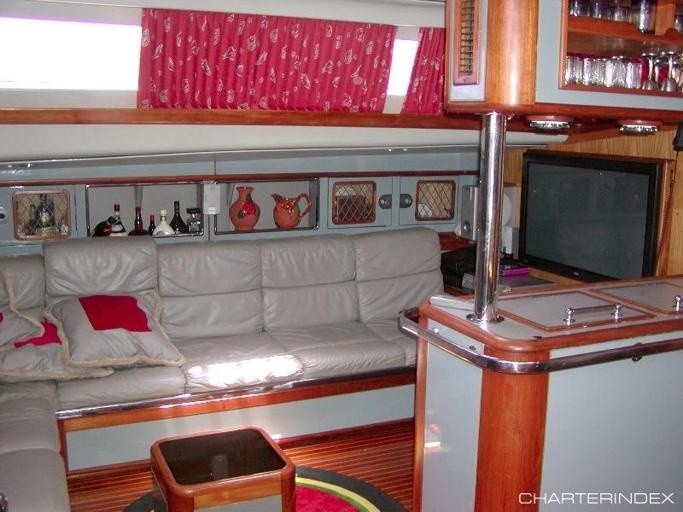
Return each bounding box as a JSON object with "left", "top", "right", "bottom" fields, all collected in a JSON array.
[{"left": 228, "top": 186, "right": 261, "bottom": 232}]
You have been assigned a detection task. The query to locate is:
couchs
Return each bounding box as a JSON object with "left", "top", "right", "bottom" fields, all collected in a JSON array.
[
  {"left": 0, "top": 378, "right": 75, "bottom": 512},
  {"left": 43, "top": 235, "right": 453, "bottom": 476}
]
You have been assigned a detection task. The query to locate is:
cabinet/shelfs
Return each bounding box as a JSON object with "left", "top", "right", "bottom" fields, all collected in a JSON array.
[
  {"left": 319, "top": 176, "right": 462, "bottom": 233},
  {"left": 445, "top": 0, "right": 536, "bottom": 118},
  {"left": 536, "top": 0, "right": 682, "bottom": 122},
  {"left": 0, "top": 186, "right": 87, "bottom": 254}
]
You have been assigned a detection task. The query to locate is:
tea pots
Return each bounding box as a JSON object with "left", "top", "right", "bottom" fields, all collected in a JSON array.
[{"left": 270, "top": 191, "right": 313, "bottom": 229}]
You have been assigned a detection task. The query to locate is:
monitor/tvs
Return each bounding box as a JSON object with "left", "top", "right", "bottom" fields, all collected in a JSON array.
[{"left": 517, "top": 149, "right": 664, "bottom": 284}]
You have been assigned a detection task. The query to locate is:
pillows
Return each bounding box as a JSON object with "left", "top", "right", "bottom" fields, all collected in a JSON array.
[
  {"left": 0, "top": 254, "right": 115, "bottom": 387},
  {"left": 44, "top": 289, "right": 186, "bottom": 370}
]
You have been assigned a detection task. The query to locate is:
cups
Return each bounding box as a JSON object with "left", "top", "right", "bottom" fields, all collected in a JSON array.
[
  {"left": 566, "top": 1, "right": 658, "bottom": 38},
  {"left": 562, "top": 54, "right": 642, "bottom": 91}
]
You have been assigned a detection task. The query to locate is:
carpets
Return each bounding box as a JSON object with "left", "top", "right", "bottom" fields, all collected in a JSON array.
[{"left": 120, "top": 466, "right": 409, "bottom": 511}]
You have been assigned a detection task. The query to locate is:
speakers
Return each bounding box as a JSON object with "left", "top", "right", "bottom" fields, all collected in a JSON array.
[
  {"left": 460, "top": 183, "right": 517, "bottom": 241},
  {"left": 499, "top": 226, "right": 517, "bottom": 257}
]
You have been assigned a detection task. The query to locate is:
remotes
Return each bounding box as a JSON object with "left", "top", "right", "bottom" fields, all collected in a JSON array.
[{"left": 429, "top": 294, "right": 473, "bottom": 310}]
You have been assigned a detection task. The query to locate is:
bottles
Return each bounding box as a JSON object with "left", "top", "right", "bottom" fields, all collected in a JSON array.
[
  {"left": 88, "top": 201, "right": 201, "bottom": 236},
  {"left": 24, "top": 192, "right": 58, "bottom": 236}
]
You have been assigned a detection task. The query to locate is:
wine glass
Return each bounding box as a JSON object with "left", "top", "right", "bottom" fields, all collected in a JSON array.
[{"left": 642, "top": 50, "right": 683, "bottom": 94}]
